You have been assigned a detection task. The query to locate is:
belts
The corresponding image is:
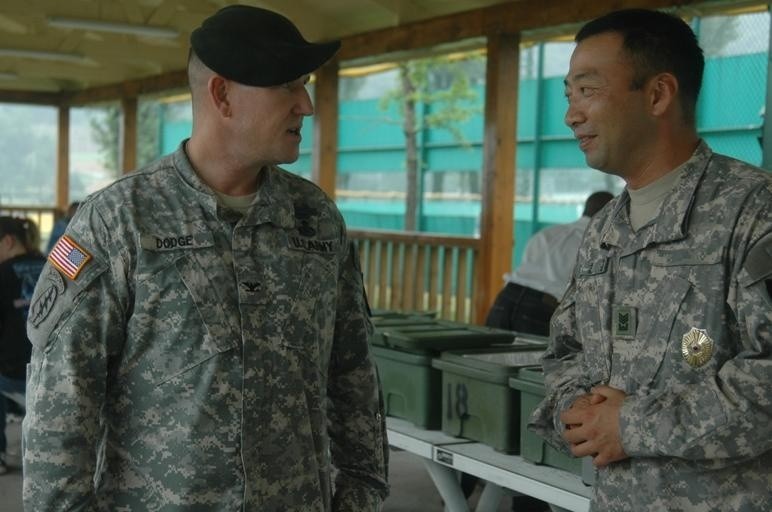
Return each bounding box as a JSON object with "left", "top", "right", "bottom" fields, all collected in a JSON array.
[{"left": 502, "top": 282, "right": 560, "bottom": 305}]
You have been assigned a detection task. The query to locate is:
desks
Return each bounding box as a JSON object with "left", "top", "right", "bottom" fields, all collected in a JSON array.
[{"left": 384, "top": 417, "right": 595, "bottom": 512}]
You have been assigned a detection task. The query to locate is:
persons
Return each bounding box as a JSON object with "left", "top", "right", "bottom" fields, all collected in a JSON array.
[
  {"left": 485, "top": 191, "right": 614, "bottom": 337},
  {"left": 0, "top": 215, "right": 49, "bottom": 475},
  {"left": 47, "top": 202, "right": 79, "bottom": 253},
  {"left": 22, "top": 5, "right": 390, "bottom": 511},
  {"left": 525, "top": 6, "right": 771, "bottom": 512}
]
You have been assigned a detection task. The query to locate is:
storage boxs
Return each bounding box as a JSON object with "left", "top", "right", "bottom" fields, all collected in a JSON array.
[{"left": 366, "top": 303, "right": 584, "bottom": 474}]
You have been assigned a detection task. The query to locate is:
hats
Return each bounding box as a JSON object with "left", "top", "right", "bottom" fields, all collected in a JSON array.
[{"left": 189, "top": 4, "right": 345, "bottom": 86}]
